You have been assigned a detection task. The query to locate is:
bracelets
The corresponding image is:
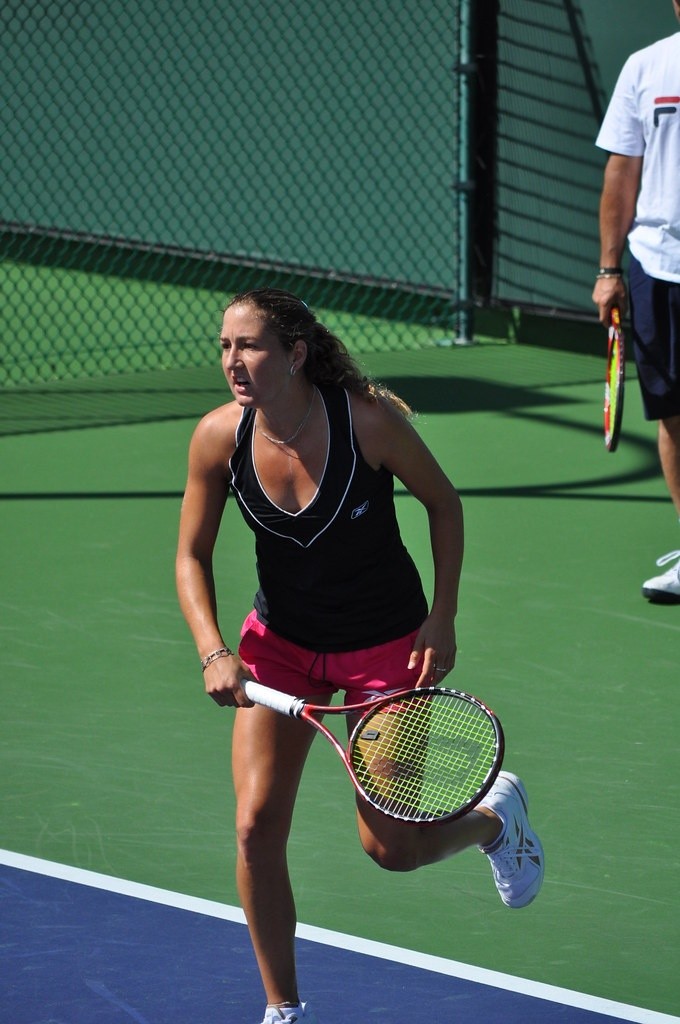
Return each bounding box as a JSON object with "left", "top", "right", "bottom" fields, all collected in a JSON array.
[
  {"left": 201, "top": 647, "right": 234, "bottom": 672},
  {"left": 597, "top": 267, "right": 625, "bottom": 278}
]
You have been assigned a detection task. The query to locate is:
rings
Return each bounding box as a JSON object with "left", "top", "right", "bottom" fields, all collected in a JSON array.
[{"left": 434, "top": 667, "right": 446, "bottom": 671}]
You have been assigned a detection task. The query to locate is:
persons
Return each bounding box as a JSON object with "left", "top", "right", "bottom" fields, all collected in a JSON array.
[
  {"left": 176, "top": 288, "right": 544, "bottom": 1024},
  {"left": 595, "top": 0, "right": 680, "bottom": 601}
]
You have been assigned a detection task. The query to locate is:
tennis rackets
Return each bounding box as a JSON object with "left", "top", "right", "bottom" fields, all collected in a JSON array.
[
  {"left": 601, "top": 304, "right": 628, "bottom": 452},
  {"left": 238, "top": 666, "right": 506, "bottom": 825}
]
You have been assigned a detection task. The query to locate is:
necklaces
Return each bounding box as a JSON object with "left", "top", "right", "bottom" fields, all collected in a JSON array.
[{"left": 255, "top": 385, "right": 317, "bottom": 444}]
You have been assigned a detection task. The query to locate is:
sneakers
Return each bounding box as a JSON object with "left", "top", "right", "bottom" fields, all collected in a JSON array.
[
  {"left": 474, "top": 770, "right": 545, "bottom": 909},
  {"left": 260, "top": 1001, "right": 306, "bottom": 1024},
  {"left": 641, "top": 550, "right": 680, "bottom": 602}
]
